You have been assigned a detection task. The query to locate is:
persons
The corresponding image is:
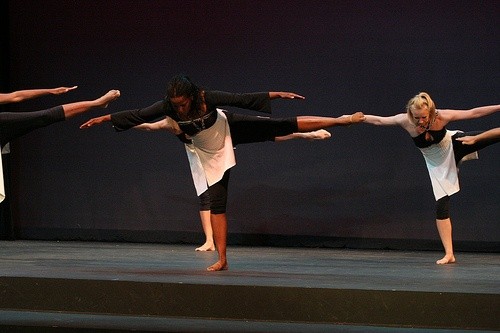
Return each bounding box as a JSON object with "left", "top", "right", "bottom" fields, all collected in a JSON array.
[
  {"left": 78, "top": 73, "right": 368, "bottom": 271},
  {"left": 455, "top": 125, "right": 500, "bottom": 146},
  {"left": 0, "top": 84, "right": 81, "bottom": 105},
  {"left": 0, "top": 88, "right": 121, "bottom": 205},
  {"left": 130, "top": 114, "right": 332, "bottom": 252},
  {"left": 334, "top": 90, "right": 500, "bottom": 265}
]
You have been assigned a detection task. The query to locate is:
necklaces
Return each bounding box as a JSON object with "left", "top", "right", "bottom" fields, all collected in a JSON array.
[
  {"left": 185, "top": 107, "right": 207, "bottom": 132},
  {"left": 420, "top": 121, "right": 432, "bottom": 139}
]
